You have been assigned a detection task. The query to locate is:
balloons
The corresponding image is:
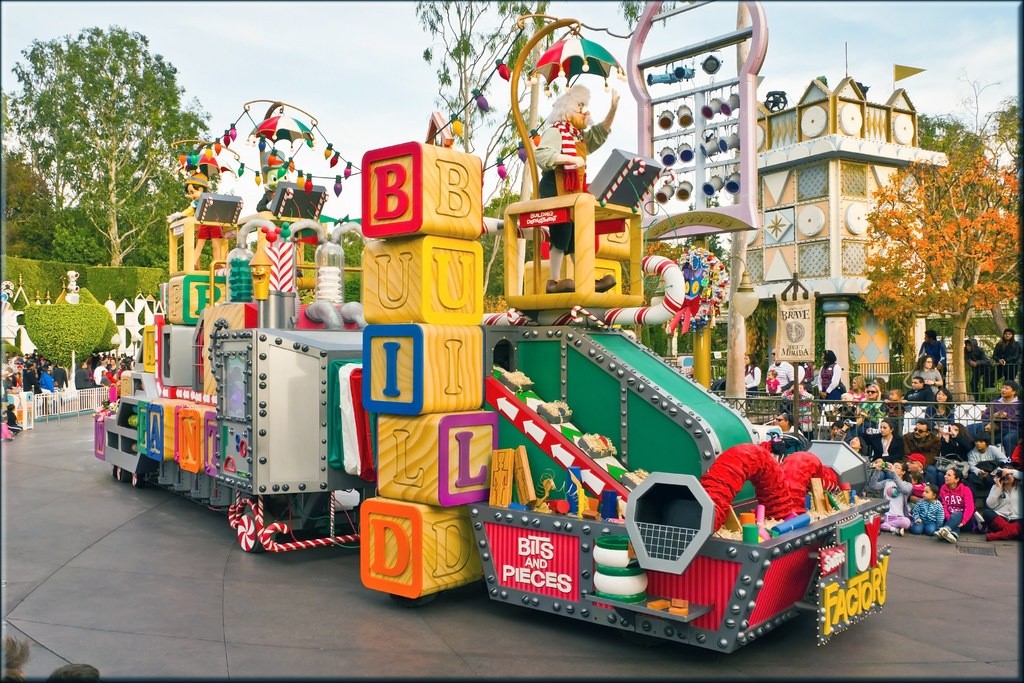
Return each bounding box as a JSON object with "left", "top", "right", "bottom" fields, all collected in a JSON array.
[{"left": 793, "top": 366, "right": 805, "bottom": 383}]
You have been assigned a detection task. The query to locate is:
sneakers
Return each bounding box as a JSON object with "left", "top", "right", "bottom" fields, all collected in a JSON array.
[
  {"left": 939, "top": 527, "right": 956, "bottom": 543},
  {"left": 934, "top": 530, "right": 945, "bottom": 539},
  {"left": 893, "top": 527, "right": 904, "bottom": 536}
]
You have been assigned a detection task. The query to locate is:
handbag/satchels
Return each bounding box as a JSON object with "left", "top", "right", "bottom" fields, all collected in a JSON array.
[
  {"left": 934, "top": 455, "right": 970, "bottom": 479},
  {"left": 836, "top": 382, "right": 846, "bottom": 393}
]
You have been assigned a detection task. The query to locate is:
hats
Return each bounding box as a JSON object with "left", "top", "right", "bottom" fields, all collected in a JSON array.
[{"left": 905, "top": 453, "right": 926, "bottom": 465}]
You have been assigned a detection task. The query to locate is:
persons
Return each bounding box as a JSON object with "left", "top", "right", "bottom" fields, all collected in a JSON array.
[
  {"left": 766, "top": 347, "right": 794, "bottom": 425},
  {"left": 256, "top": 149, "right": 303, "bottom": 277},
  {"left": 965, "top": 339, "right": 993, "bottom": 401},
  {"left": 75, "top": 352, "right": 135, "bottom": 412},
  {"left": 818, "top": 350, "right": 842, "bottom": 401},
  {"left": 534, "top": 85, "right": 620, "bottom": 293},
  {"left": 745, "top": 353, "right": 762, "bottom": 397},
  {"left": 912, "top": 356, "right": 943, "bottom": 397},
  {"left": 925, "top": 388, "right": 955, "bottom": 433},
  {"left": 1, "top": 348, "right": 68, "bottom": 440},
  {"left": 965, "top": 381, "right": 1021, "bottom": 462},
  {"left": 826, "top": 393, "right": 1021, "bottom": 543},
  {"left": 782, "top": 383, "right": 815, "bottom": 441},
  {"left": 167, "top": 172, "right": 223, "bottom": 270},
  {"left": 919, "top": 330, "right": 947, "bottom": 389},
  {"left": 798, "top": 361, "right": 815, "bottom": 394},
  {"left": 905, "top": 377, "right": 937, "bottom": 402},
  {"left": 773, "top": 413, "right": 807, "bottom": 460},
  {"left": 992, "top": 328, "right": 1021, "bottom": 381},
  {"left": 847, "top": 376, "right": 912, "bottom": 432}
]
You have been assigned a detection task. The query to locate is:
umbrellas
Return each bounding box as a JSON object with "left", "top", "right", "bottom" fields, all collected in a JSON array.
[
  {"left": 527, "top": 38, "right": 628, "bottom": 96},
  {"left": 246, "top": 115, "right": 317, "bottom": 152},
  {"left": 177, "top": 153, "right": 238, "bottom": 182}
]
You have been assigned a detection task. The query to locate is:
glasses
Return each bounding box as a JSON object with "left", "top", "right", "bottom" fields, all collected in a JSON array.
[
  {"left": 771, "top": 353, "right": 775, "bottom": 356},
  {"left": 912, "top": 382, "right": 918, "bottom": 384},
  {"left": 866, "top": 391, "right": 877, "bottom": 394},
  {"left": 776, "top": 418, "right": 784, "bottom": 422},
  {"left": 949, "top": 429, "right": 959, "bottom": 432},
  {"left": 906, "top": 459, "right": 914, "bottom": 464},
  {"left": 913, "top": 427, "right": 928, "bottom": 433}
]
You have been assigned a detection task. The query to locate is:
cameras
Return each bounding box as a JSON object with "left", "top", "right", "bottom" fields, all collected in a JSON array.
[{"left": 996, "top": 470, "right": 1006, "bottom": 480}]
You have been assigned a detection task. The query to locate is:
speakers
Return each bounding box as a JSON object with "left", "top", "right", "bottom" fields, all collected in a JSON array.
[
  {"left": 195, "top": 193, "right": 243, "bottom": 224},
  {"left": 270, "top": 181, "right": 326, "bottom": 219},
  {"left": 588, "top": 149, "right": 662, "bottom": 207}
]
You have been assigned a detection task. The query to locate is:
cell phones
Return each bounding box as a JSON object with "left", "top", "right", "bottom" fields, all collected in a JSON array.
[
  {"left": 882, "top": 462, "right": 887, "bottom": 468},
  {"left": 944, "top": 425, "right": 948, "bottom": 432}
]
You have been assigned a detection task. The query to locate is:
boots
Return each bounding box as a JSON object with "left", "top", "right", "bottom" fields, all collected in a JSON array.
[
  {"left": 986, "top": 521, "right": 1022, "bottom": 541},
  {"left": 991, "top": 516, "right": 1011, "bottom": 530}
]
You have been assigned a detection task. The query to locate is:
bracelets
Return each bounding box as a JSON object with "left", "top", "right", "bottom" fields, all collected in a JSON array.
[{"left": 933, "top": 381, "right": 936, "bottom": 385}]
